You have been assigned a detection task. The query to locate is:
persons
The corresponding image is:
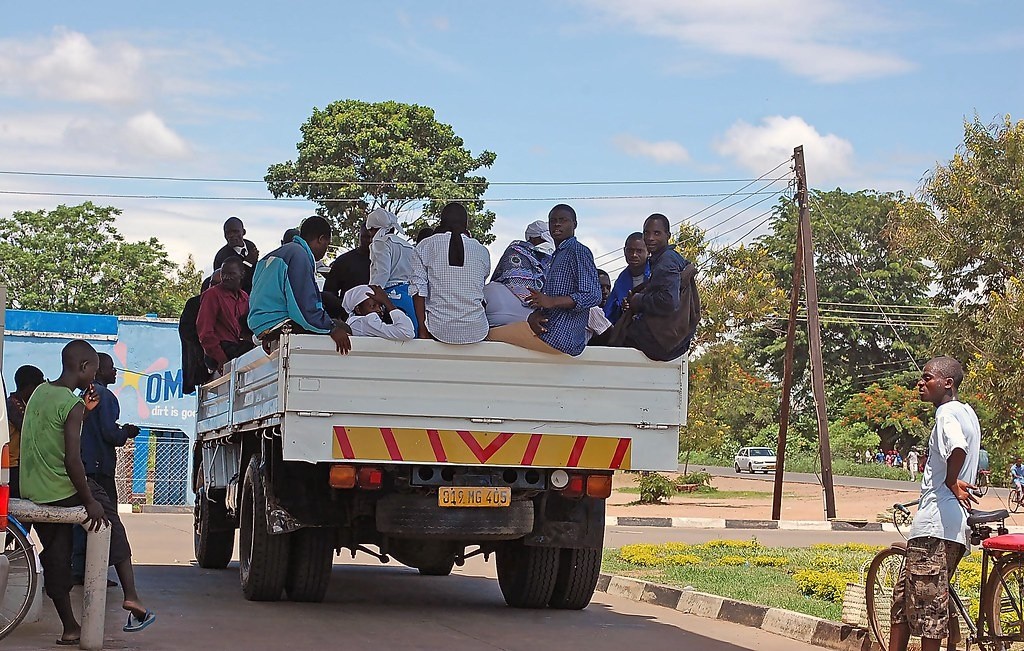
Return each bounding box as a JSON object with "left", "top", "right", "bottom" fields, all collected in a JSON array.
[
  {"left": 179, "top": 200, "right": 702, "bottom": 396},
  {"left": 1010, "top": 457, "right": 1024, "bottom": 508},
  {"left": 856, "top": 445, "right": 929, "bottom": 482},
  {"left": 890, "top": 357, "right": 980, "bottom": 651},
  {"left": 69, "top": 353, "right": 141, "bottom": 587},
  {"left": 5, "top": 364, "right": 45, "bottom": 554},
  {"left": 20, "top": 339, "right": 155, "bottom": 646},
  {"left": 978, "top": 443, "right": 989, "bottom": 470}
]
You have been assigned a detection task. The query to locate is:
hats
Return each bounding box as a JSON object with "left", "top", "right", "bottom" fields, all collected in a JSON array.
[{"left": 911, "top": 446, "right": 916, "bottom": 449}]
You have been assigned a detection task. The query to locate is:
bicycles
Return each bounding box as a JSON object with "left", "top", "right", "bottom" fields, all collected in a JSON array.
[
  {"left": 1008, "top": 486, "right": 1024, "bottom": 513},
  {"left": 977, "top": 470, "right": 990, "bottom": 495},
  {"left": 0, "top": 512, "right": 39, "bottom": 639},
  {"left": 864, "top": 492, "right": 1020, "bottom": 651}
]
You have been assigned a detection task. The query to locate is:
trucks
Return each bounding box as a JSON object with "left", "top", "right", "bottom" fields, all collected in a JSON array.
[{"left": 192, "top": 327, "right": 691, "bottom": 608}]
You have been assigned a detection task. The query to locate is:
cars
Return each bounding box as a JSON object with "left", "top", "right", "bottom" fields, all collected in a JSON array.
[
  {"left": 0, "top": 380, "right": 10, "bottom": 531},
  {"left": 734, "top": 447, "right": 775, "bottom": 474}
]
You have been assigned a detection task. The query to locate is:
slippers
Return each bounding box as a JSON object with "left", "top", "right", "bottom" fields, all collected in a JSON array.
[
  {"left": 56, "top": 639, "right": 80, "bottom": 645},
  {"left": 107, "top": 580, "right": 118, "bottom": 586},
  {"left": 122, "top": 608, "right": 156, "bottom": 632}
]
